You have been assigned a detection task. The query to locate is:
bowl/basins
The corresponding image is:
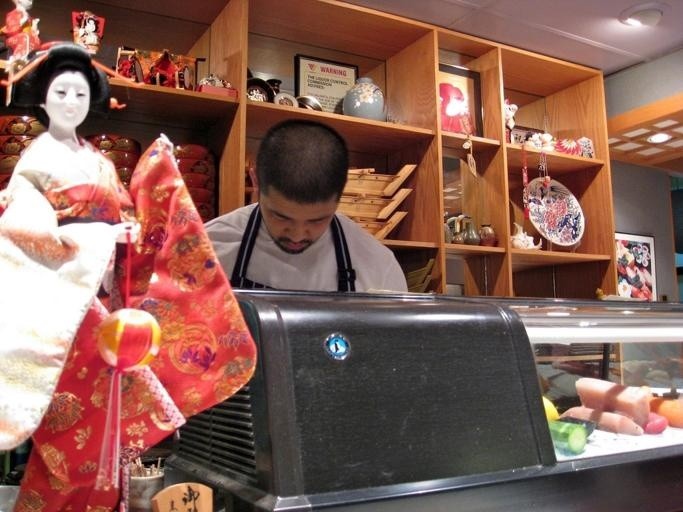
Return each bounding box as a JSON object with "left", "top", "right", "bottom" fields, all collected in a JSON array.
[{"left": 1, "top": 115, "right": 217, "bottom": 227}]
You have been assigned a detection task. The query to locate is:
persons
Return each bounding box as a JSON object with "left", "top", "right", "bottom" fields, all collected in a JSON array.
[
  {"left": 74, "top": 13, "right": 100, "bottom": 53},
  {"left": 201, "top": 118, "right": 408, "bottom": 294},
  {"left": 0, "top": 0, "right": 41, "bottom": 62},
  {"left": 0, "top": 40, "right": 259, "bottom": 511}
]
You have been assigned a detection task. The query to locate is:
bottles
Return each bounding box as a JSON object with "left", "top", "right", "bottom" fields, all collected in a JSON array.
[{"left": 444, "top": 214, "right": 496, "bottom": 248}]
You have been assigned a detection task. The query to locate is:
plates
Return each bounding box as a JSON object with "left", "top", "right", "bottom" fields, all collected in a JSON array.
[
  {"left": 521, "top": 176, "right": 586, "bottom": 248},
  {"left": 247, "top": 65, "right": 325, "bottom": 113}
]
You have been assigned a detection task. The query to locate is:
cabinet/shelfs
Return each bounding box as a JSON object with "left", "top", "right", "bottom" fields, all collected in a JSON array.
[
  {"left": 163, "top": 293, "right": 681, "bottom": 512},
  {"left": 0, "top": 1, "right": 682, "bottom": 296}
]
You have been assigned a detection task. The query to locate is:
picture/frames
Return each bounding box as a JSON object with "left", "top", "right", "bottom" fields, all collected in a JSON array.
[{"left": 614, "top": 231, "right": 657, "bottom": 301}]
